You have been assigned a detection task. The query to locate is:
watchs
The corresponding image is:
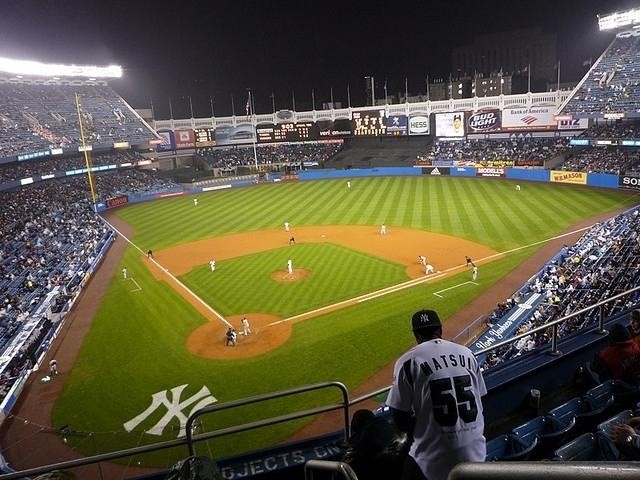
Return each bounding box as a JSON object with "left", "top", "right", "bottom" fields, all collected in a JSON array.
[{"left": 625, "top": 433, "right": 637, "bottom": 446}]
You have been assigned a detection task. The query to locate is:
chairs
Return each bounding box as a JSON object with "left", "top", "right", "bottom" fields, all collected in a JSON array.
[
  {"left": 0, "top": 201, "right": 111, "bottom": 403},
  {"left": 370, "top": 201, "right": 640, "bottom": 461},
  {"left": 0, "top": 30, "right": 640, "bottom": 200}
]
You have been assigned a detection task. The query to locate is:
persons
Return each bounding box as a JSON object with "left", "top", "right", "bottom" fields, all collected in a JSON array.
[
  {"left": 193, "top": 198, "right": 199, "bottom": 206},
  {"left": 471, "top": 265, "right": 478, "bottom": 281},
  {"left": 424, "top": 264, "right": 434, "bottom": 275},
  {"left": 414, "top": 120, "right": 640, "bottom": 183},
  {"left": 231, "top": 329, "right": 238, "bottom": 341},
  {"left": 284, "top": 221, "right": 289, "bottom": 231},
  {"left": 343, "top": 408, "right": 432, "bottom": 479},
  {"left": 347, "top": 180, "right": 350, "bottom": 188},
  {"left": 515, "top": 184, "right": 520, "bottom": 192},
  {"left": 556, "top": 31, "right": 640, "bottom": 119},
  {"left": 208, "top": 258, "right": 217, "bottom": 271},
  {"left": 288, "top": 237, "right": 296, "bottom": 244},
  {"left": 478, "top": 208, "right": 640, "bottom": 384},
  {"left": 386, "top": 308, "right": 489, "bottom": 479},
  {"left": 287, "top": 258, "right": 293, "bottom": 274},
  {"left": 190, "top": 138, "right": 346, "bottom": 173},
  {"left": 1, "top": 74, "right": 179, "bottom": 410},
  {"left": 417, "top": 255, "right": 427, "bottom": 265},
  {"left": 605, "top": 414, "right": 640, "bottom": 456},
  {"left": 241, "top": 316, "right": 251, "bottom": 336},
  {"left": 464, "top": 255, "right": 476, "bottom": 267},
  {"left": 380, "top": 224, "right": 386, "bottom": 236},
  {"left": 225, "top": 327, "right": 236, "bottom": 346}
]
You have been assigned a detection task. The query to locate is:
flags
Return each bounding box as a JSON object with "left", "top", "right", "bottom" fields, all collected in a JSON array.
[{"left": 245, "top": 98, "right": 251, "bottom": 116}]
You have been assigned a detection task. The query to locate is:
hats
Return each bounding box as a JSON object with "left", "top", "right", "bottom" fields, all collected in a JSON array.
[{"left": 411, "top": 309, "right": 443, "bottom": 330}]
